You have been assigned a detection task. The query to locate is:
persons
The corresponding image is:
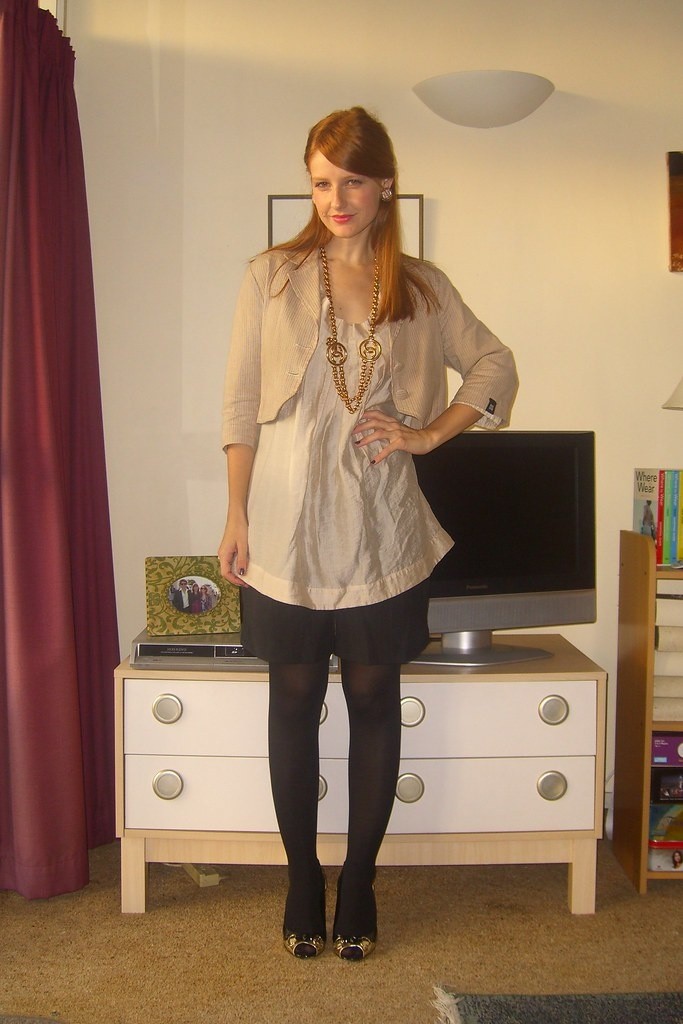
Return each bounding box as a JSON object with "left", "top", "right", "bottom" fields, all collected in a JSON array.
[
  {"left": 170, "top": 580, "right": 217, "bottom": 613},
  {"left": 216, "top": 108, "right": 519, "bottom": 960},
  {"left": 672, "top": 851, "right": 682, "bottom": 869}
]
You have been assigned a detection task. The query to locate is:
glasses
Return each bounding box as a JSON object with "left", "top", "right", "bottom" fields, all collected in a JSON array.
[
  {"left": 201, "top": 588, "right": 206, "bottom": 590},
  {"left": 181, "top": 582, "right": 186, "bottom": 585}
]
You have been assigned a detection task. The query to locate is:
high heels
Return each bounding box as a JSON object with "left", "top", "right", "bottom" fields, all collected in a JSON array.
[
  {"left": 284, "top": 868, "right": 328, "bottom": 958},
  {"left": 333, "top": 865, "right": 379, "bottom": 960}
]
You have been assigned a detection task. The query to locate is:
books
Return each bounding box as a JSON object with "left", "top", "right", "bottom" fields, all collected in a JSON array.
[{"left": 633, "top": 468, "right": 683, "bottom": 565}]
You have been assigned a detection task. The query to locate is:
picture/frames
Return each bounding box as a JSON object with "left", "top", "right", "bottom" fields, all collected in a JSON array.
[
  {"left": 144, "top": 556, "right": 242, "bottom": 636},
  {"left": 267, "top": 194, "right": 424, "bottom": 261}
]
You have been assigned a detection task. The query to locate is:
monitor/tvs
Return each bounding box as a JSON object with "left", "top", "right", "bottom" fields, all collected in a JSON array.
[{"left": 409, "top": 430, "right": 598, "bottom": 666}]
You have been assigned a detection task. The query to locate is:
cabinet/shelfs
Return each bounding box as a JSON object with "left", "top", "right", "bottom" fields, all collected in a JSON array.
[
  {"left": 113, "top": 633, "right": 610, "bottom": 914},
  {"left": 612, "top": 529, "right": 683, "bottom": 890}
]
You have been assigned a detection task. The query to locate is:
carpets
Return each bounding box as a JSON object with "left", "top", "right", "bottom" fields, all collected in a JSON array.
[{"left": 430, "top": 986, "right": 683, "bottom": 1024}]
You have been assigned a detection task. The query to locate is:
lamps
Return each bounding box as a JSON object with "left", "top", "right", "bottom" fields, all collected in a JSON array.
[{"left": 412, "top": 68, "right": 555, "bottom": 128}]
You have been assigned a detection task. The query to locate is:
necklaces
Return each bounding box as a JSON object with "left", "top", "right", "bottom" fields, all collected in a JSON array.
[{"left": 319, "top": 246, "right": 382, "bottom": 414}]
